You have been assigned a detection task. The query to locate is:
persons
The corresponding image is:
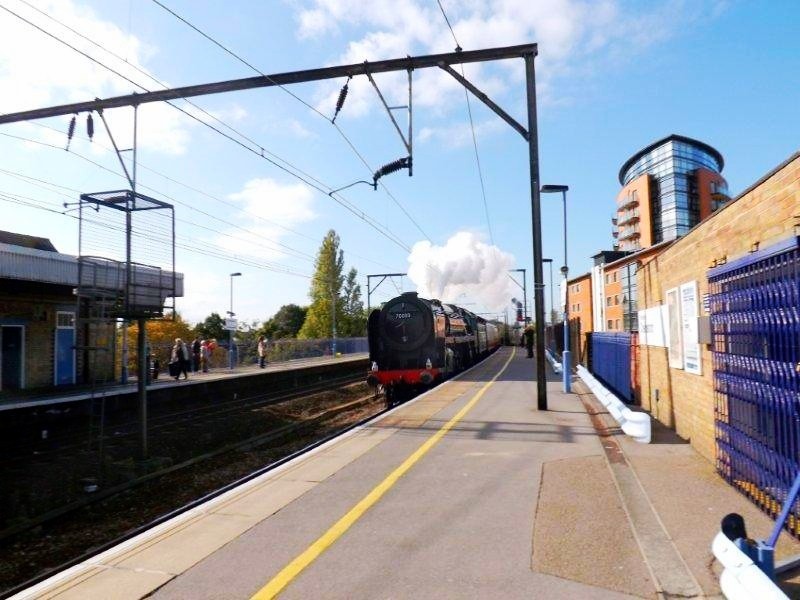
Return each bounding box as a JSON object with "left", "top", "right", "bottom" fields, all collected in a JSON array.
[
  {"left": 257, "top": 335, "right": 266, "bottom": 368},
  {"left": 200, "top": 339, "right": 216, "bottom": 373},
  {"left": 191, "top": 336, "right": 200, "bottom": 374},
  {"left": 170, "top": 337, "right": 189, "bottom": 381},
  {"left": 525, "top": 325, "right": 535, "bottom": 358}
]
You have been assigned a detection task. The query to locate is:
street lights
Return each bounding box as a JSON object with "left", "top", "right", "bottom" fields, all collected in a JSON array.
[
  {"left": 507, "top": 268, "right": 527, "bottom": 329},
  {"left": 542, "top": 258, "right": 556, "bottom": 358},
  {"left": 228, "top": 272, "right": 242, "bottom": 371},
  {"left": 541, "top": 183, "right": 574, "bottom": 395},
  {"left": 331, "top": 292, "right": 341, "bottom": 359}
]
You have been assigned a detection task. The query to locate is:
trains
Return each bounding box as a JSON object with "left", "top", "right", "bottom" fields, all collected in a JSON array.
[{"left": 364, "top": 290, "right": 513, "bottom": 409}]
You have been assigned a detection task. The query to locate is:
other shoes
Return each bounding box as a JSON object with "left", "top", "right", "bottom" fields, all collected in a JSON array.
[
  {"left": 183, "top": 376, "right": 187, "bottom": 380},
  {"left": 260, "top": 366, "right": 265, "bottom": 369},
  {"left": 262, "top": 365, "right": 266, "bottom": 367},
  {"left": 174, "top": 377, "right": 179, "bottom": 380}
]
